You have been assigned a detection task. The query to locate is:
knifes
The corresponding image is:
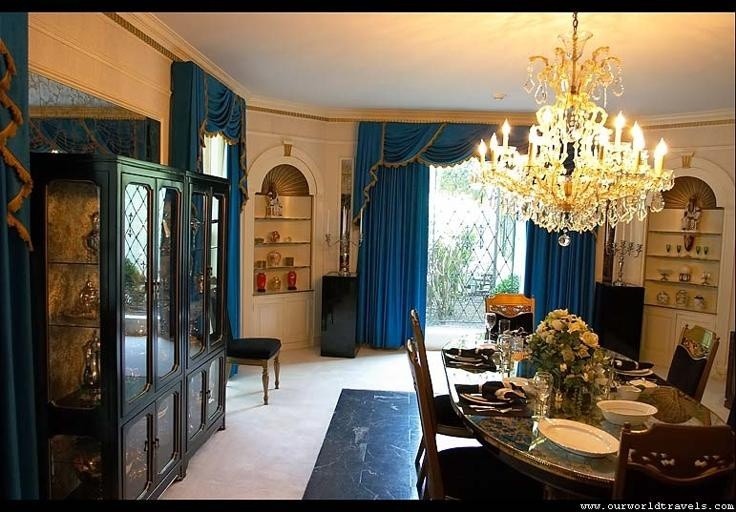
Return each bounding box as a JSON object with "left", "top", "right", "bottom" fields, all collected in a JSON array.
[{"left": 469, "top": 404, "right": 523, "bottom": 413}]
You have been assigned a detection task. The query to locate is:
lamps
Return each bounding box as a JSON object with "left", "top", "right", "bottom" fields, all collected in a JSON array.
[{"left": 472, "top": 13, "right": 675, "bottom": 247}]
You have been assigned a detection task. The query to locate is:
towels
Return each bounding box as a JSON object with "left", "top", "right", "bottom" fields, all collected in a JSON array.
[
  {"left": 610, "top": 357, "right": 654, "bottom": 371},
  {"left": 482, "top": 381, "right": 527, "bottom": 405},
  {"left": 446, "top": 347, "right": 495, "bottom": 357}
]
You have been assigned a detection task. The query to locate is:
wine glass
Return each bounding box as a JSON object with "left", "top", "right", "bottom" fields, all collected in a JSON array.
[
  {"left": 485, "top": 313, "right": 496, "bottom": 342},
  {"left": 533, "top": 372, "right": 553, "bottom": 417},
  {"left": 666, "top": 244, "right": 671, "bottom": 256},
  {"left": 696, "top": 246, "right": 700, "bottom": 258},
  {"left": 704, "top": 247, "right": 708, "bottom": 258},
  {"left": 499, "top": 319, "right": 510, "bottom": 334},
  {"left": 677, "top": 245, "right": 681, "bottom": 256}
]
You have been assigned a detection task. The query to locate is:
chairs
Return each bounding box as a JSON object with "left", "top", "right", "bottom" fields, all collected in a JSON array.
[
  {"left": 666, "top": 324, "right": 721, "bottom": 404},
  {"left": 407, "top": 339, "right": 532, "bottom": 500},
  {"left": 485, "top": 294, "right": 535, "bottom": 342},
  {"left": 410, "top": 309, "right": 476, "bottom": 466},
  {"left": 607, "top": 421, "right": 736, "bottom": 502},
  {"left": 223, "top": 303, "right": 282, "bottom": 406}
]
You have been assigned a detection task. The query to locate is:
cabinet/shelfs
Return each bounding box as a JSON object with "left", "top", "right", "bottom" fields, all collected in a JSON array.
[
  {"left": 184, "top": 168, "right": 232, "bottom": 479},
  {"left": 27, "top": 151, "right": 187, "bottom": 501},
  {"left": 642, "top": 157, "right": 735, "bottom": 382},
  {"left": 239, "top": 143, "right": 316, "bottom": 351}
]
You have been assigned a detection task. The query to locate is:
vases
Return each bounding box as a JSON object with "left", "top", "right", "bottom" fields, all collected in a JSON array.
[{"left": 544, "top": 368, "right": 597, "bottom": 419}]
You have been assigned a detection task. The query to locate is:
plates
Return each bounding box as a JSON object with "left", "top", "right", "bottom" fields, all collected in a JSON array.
[
  {"left": 445, "top": 353, "right": 482, "bottom": 362},
  {"left": 464, "top": 393, "right": 488, "bottom": 401},
  {"left": 629, "top": 379, "right": 657, "bottom": 395},
  {"left": 537, "top": 419, "right": 619, "bottom": 458},
  {"left": 615, "top": 370, "right": 654, "bottom": 377},
  {"left": 459, "top": 393, "right": 508, "bottom": 406},
  {"left": 616, "top": 368, "right": 650, "bottom": 374}
]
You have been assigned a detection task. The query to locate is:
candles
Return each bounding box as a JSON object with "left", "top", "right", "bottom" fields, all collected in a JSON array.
[
  {"left": 606, "top": 219, "right": 608, "bottom": 241},
  {"left": 614, "top": 224, "right": 617, "bottom": 244},
  {"left": 326, "top": 208, "right": 329, "bottom": 234},
  {"left": 623, "top": 223, "right": 625, "bottom": 241},
  {"left": 640, "top": 222, "right": 642, "bottom": 244},
  {"left": 630, "top": 221, "right": 632, "bottom": 241},
  {"left": 342, "top": 206, "right": 346, "bottom": 235},
  {"left": 360, "top": 208, "right": 363, "bottom": 235}
]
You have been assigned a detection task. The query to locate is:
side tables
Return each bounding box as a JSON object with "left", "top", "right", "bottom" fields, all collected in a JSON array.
[
  {"left": 592, "top": 280, "right": 644, "bottom": 364},
  {"left": 322, "top": 271, "right": 361, "bottom": 358}
]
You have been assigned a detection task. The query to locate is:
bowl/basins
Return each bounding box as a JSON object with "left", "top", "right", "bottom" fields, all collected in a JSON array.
[
  {"left": 596, "top": 400, "right": 657, "bottom": 426},
  {"left": 617, "top": 386, "right": 642, "bottom": 401}
]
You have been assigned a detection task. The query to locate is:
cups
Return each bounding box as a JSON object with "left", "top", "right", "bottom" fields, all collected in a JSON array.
[
  {"left": 500, "top": 337, "right": 511, "bottom": 352},
  {"left": 513, "top": 337, "right": 524, "bottom": 351}
]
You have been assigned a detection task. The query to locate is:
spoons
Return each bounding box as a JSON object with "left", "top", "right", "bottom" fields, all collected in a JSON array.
[
  {"left": 475, "top": 407, "right": 512, "bottom": 414},
  {"left": 449, "top": 361, "right": 484, "bottom": 367}
]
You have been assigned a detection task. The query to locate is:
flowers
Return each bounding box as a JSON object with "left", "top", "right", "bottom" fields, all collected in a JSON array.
[{"left": 521, "top": 308, "right": 606, "bottom": 423}]
